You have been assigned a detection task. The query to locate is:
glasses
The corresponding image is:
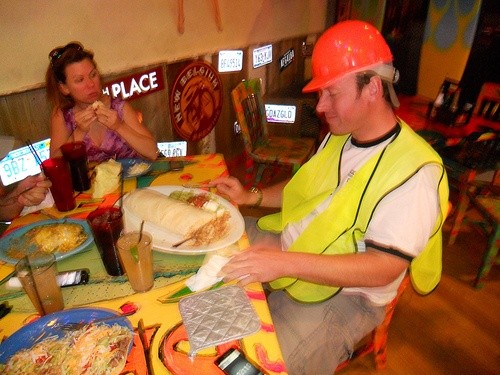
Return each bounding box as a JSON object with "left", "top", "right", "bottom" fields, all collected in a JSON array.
[{"left": 49, "top": 41, "right": 83, "bottom": 64}]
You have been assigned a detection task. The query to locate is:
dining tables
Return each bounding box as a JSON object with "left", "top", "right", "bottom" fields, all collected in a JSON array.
[{"left": 0, "top": 154, "right": 289, "bottom": 375}]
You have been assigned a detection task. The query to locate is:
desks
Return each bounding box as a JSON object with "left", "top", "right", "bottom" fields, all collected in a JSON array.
[{"left": 431, "top": 125, "right": 500, "bottom": 247}]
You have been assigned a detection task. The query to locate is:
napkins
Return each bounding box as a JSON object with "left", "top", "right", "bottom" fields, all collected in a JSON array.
[
  {"left": 19, "top": 187, "right": 54, "bottom": 217},
  {"left": 185, "top": 255, "right": 238, "bottom": 293}
]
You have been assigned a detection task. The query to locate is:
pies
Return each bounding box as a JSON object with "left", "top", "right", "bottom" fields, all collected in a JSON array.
[{"left": 123, "top": 187, "right": 218, "bottom": 235}]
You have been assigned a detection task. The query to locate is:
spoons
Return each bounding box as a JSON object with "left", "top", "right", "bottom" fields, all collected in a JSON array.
[{"left": 0, "top": 186, "right": 35, "bottom": 207}]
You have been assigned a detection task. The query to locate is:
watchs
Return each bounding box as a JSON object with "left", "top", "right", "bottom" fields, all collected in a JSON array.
[{"left": 248, "top": 186, "right": 263, "bottom": 209}]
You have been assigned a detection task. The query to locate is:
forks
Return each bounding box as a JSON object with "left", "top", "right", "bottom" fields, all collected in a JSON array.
[{"left": 60, "top": 311, "right": 136, "bottom": 330}]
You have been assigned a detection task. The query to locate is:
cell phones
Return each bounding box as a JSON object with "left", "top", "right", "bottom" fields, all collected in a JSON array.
[
  {"left": 58, "top": 269, "right": 89, "bottom": 287},
  {"left": 214, "top": 347, "right": 266, "bottom": 375}
]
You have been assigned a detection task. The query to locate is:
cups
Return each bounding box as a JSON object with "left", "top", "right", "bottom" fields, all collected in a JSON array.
[
  {"left": 116, "top": 231, "right": 155, "bottom": 291},
  {"left": 60, "top": 140, "right": 91, "bottom": 191},
  {"left": 15, "top": 250, "right": 65, "bottom": 315},
  {"left": 40, "top": 157, "right": 76, "bottom": 211},
  {"left": 86, "top": 205, "right": 126, "bottom": 276}
]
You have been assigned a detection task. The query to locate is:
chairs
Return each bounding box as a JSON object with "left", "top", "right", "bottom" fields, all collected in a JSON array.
[
  {"left": 231, "top": 77, "right": 316, "bottom": 188},
  {"left": 467, "top": 83, "right": 500, "bottom": 135},
  {"left": 446, "top": 193, "right": 500, "bottom": 290},
  {"left": 336, "top": 269, "right": 410, "bottom": 370}
]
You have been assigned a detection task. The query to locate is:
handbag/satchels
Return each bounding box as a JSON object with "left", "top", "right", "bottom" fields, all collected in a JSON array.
[{"left": 178, "top": 285, "right": 261, "bottom": 363}]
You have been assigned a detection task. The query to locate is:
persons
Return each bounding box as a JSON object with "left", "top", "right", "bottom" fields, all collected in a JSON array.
[
  {"left": 0, "top": 42, "right": 159, "bottom": 221},
  {"left": 210, "top": 20, "right": 449, "bottom": 375}
]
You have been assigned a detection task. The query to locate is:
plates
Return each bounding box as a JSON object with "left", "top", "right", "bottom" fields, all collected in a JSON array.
[
  {"left": 112, "top": 185, "right": 245, "bottom": 254},
  {"left": 0, "top": 307, "right": 134, "bottom": 375},
  {"left": 0, "top": 219, "right": 93, "bottom": 263},
  {"left": 116, "top": 158, "right": 153, "bottom": 178}
]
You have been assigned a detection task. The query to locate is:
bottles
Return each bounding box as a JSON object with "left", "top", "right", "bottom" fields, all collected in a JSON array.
[{"left": 427, "top": 81, "right": 465, "bottom": 126}]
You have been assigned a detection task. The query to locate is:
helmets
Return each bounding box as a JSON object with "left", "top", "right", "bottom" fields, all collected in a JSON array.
[{"left": 301, "top": 21, "right": 392, "bottom": 93}]
